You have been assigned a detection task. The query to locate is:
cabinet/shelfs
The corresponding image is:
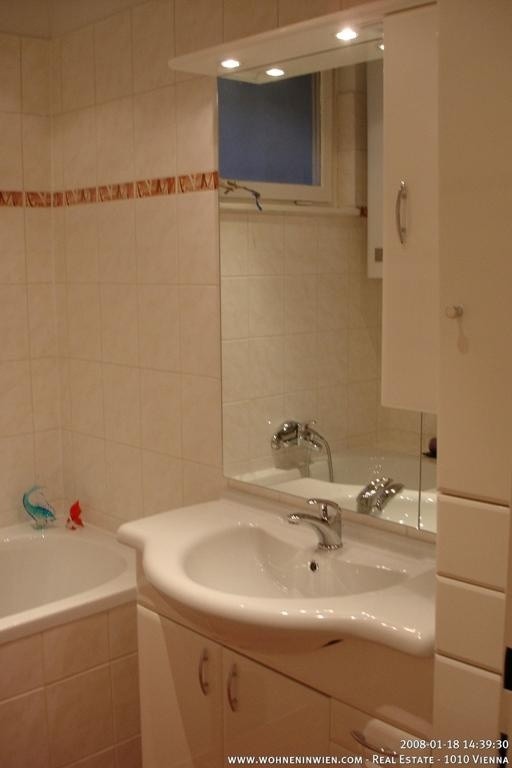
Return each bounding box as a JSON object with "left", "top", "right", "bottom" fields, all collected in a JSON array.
[
  {"left": 135, "top": 600, "right": 330, "bottom": 768},
  {"left": 435, "top": 2, "right": 510, "bottom": 767},
  {"left": 329, "top": 696, "right": 431, "bottom": 768},
  {"left": 377, "top": 0, "right": 439, "bottom": 419}
]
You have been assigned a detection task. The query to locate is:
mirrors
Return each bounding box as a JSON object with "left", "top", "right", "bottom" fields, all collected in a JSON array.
[{"left": 214, "top": 0, "right": 439, "bottom": 540}]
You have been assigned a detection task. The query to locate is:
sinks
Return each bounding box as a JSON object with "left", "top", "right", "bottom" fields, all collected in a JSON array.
[
  {"left": 234, "top": 445, "right": 437, "bottom": 532},
  {"left": 114, "top": 497, "right": 437, "bottom": 659}
]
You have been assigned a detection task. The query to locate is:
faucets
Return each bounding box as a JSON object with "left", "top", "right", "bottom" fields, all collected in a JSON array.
[
  {"left": 287, "top": 497, "right": 343, "bottom": 551},
  {"left": 355, "top": 477, "right": 405, "bottom": 517}
]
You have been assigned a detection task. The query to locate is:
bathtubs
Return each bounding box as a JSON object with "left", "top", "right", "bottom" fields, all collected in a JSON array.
[{"left": 0, "top": 516, "right": 139, "bottom": 647}]
